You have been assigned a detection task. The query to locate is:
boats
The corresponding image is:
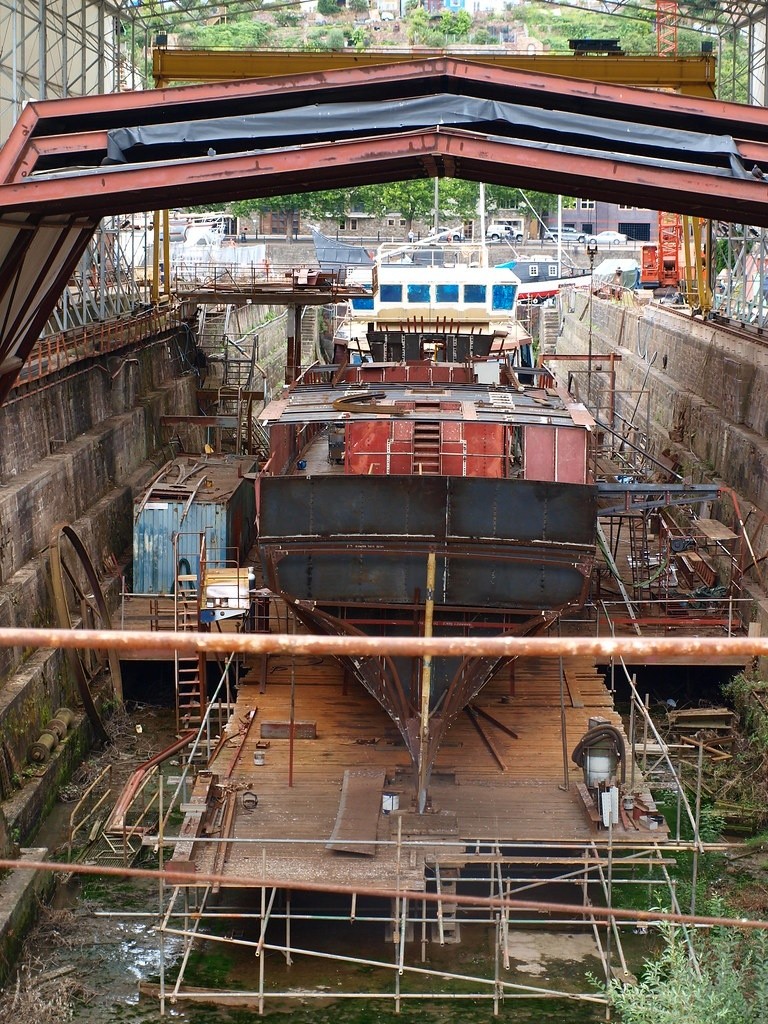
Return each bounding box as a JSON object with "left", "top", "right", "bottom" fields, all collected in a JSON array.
[{"left": 241, "top": 315, "right": 600, "bottom": 811}]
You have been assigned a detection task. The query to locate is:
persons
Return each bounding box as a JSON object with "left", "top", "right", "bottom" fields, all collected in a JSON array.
[
  {"left": 407, "top": 229, "right": 414, "bottom": 243},
  {"left": 447, "top": 233, "right": 452, "bottom": 243},
  {"left": 508, "top": 227, "right": 514, "bottom": 244},
  {"left": 240, "top": 227, "right": 248, "bottom": 243}
]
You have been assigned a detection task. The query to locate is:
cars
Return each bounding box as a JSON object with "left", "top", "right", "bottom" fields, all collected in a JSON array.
[{"left": 164, "top": 208, "right": 628, "bottom": 245}]
